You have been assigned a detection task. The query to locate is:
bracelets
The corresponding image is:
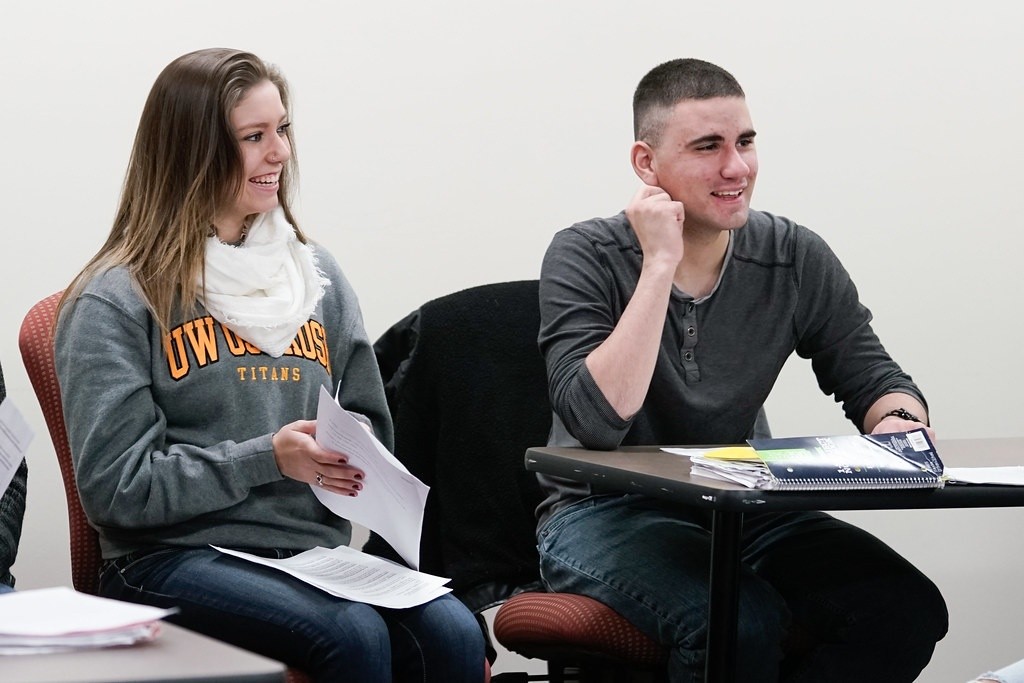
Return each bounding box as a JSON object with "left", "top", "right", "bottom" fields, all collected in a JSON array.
[{"left": 881, "top": 407, "right": 921, "bottom": 423}]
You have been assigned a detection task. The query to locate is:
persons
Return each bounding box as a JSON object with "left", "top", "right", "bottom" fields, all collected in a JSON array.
[
  {"left": 50, "top": 48, "right": 486, "bottom": 683},
  {"left": 534, "top": 57, "right": 949, "bottom": 683}
]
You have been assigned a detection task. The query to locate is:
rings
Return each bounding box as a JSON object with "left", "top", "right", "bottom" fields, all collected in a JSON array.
[{"left": 315, "top": 474, "right": 323, "bottom": 485}]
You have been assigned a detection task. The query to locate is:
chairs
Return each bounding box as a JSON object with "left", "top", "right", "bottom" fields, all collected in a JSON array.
[
  {"left": 17, "top": 287, "right": 492, "bottom": 683},
  {"left": 371, "top": 279, "right": 772, "bottom": 683}
]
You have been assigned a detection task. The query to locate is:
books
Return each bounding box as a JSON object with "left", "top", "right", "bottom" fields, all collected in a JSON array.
[{"left": 689, "top": 428, "right": 946, "bottom": 491}]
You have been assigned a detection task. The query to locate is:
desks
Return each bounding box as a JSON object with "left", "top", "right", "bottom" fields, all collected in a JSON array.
[
  {"left": 521, "top": 445, "right": 1024, "bottom": 683},
  {"left": 0, "top": 619, "right": 286, "bottom": 683}
]
